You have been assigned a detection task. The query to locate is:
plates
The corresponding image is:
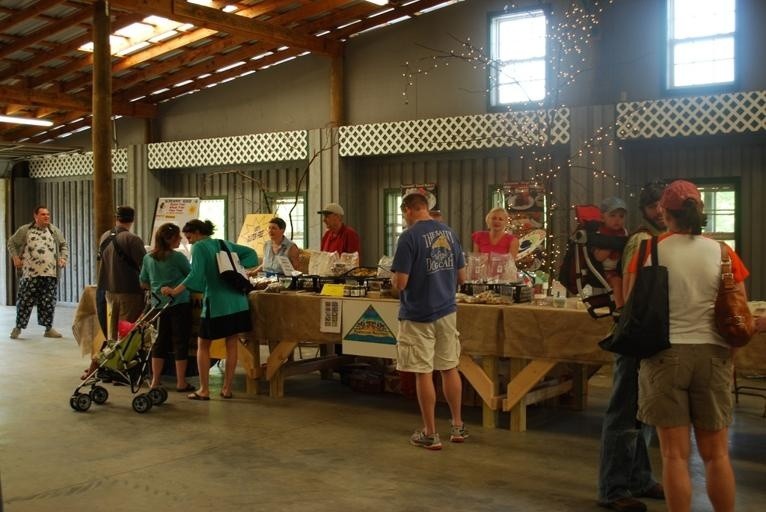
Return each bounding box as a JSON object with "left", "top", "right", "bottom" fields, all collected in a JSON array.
[
  {"left": 507, "top": 194, "right": 534, "bottom": 210},
  {"left": 403, "top": 189, "right": 436, "bottom": 210}
]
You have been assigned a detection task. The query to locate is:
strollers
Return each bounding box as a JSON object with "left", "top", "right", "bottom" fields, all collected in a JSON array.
[{"left": 71, "top": 290, "right": 175, "bottom": 414}]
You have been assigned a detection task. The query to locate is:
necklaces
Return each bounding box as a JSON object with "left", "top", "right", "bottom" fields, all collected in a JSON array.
[{"left": 269, "top": 241, "right": 281, "bottom": 256}]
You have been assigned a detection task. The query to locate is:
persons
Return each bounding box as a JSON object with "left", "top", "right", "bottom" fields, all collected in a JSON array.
[
  {"left": 317, "top": 202, "right": 361, "bottom": 264},
  {"left": 79, "top": 319, "right": 132, "bottom": 381},
  {"left": 159, "top": 219, "right": 258, "bottom": 400},
  {"left": 594, "top": 196, "right": 628, "bottom": 318},
  {"left": 139, "top": 222, "right": 194, "bottom": 392},
  {"left": 625, "top": 178, "right": 757, "bottom": 511},
  {"left": 6, "top": 205, "right": 69, "bottom": 339},
  {"left": 95, "top": 204, "right": 147, "bottom": 338},
  {"left": 245, "top": 218, "right": 301, "bottom": 279},
  {"left": 387, "top": 191, "right": 472, "bottom": 450},
  {"left": 597, "top": 182, "right": 670, "bottom": 510},
  {"left": 471, "top": 207, "right": 520, "bottom": 262}
]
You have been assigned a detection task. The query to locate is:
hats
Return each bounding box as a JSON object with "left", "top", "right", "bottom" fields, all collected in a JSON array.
[
  {"left": 639, "top": 180, "right": 701, "bottom": 209},
  {"left": 601, "top": 197, "right": 627, "bottom": 213},
  {"left": 317, "top": 203, "right": 344, "bottom": 216}
]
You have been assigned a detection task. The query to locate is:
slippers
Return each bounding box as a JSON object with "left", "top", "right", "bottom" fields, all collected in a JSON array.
[{"left": 177, "top": 382, "right": 232, "bottom": 400}]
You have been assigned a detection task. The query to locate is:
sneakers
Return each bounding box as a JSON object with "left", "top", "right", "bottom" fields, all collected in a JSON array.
[
  {"left": 410, "top": 431, "right": 442, "bottom": 449},
  {"left": 450, "top": 423, "right": 470, "bottom": 442},
  {"left": 599, "top": 498, "right": 646, "bottom": 512},
  {"left": 10, "top": 328, "right": 20, "bottom": 338},
  {"left": 44, "top": 328, "right": 62, "bottom": 337},
  {"left": 633, "top": 482, "right": 665, "bottom": 498}
]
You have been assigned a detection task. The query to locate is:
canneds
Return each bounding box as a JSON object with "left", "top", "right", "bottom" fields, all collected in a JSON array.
[{"left": 343, "top": 285, "right": 366, "bottom": 297}]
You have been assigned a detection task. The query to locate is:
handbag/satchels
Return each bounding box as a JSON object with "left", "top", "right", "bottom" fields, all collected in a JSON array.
[
  {"left": 715, "top": 273, "right": 753, "bottom": 346},
  {"left": 216, "top": 251, "right": 250, "bottom": 291},
  {"left": 598, "top": 266, "right": 671, "bottom": 358}
]
